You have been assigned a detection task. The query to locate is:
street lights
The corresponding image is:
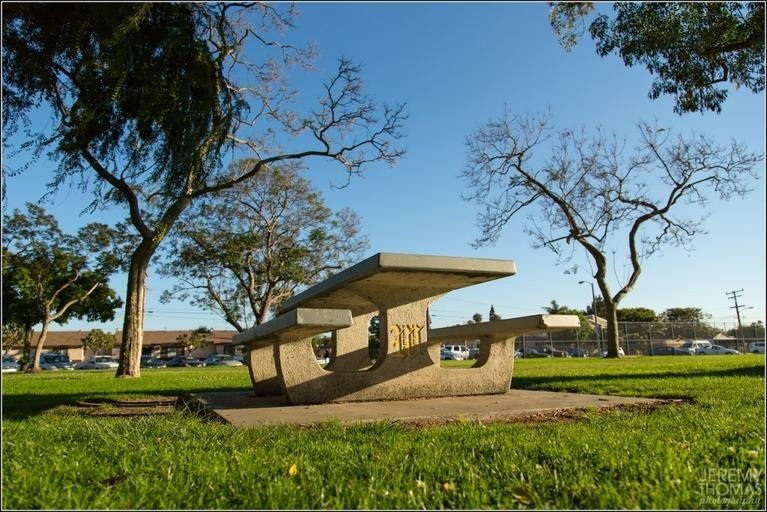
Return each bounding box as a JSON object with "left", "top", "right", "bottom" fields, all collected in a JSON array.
[
  {"left": 737, "top": 305, "right": 755, "bottom": 354},
  {"left": 578, "top": 279, "right": 602, "bottom": 355}
]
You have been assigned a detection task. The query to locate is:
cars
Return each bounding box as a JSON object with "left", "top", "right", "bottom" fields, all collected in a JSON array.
[
  {"left": 514, "top": 335, "right": 766, "bottom": 358},
  {"left": 441, "top": 341, "right": 480, "bottom": 361},
  {"left": 1, "top": 352, "right": 249, "bottom": 373}
]
